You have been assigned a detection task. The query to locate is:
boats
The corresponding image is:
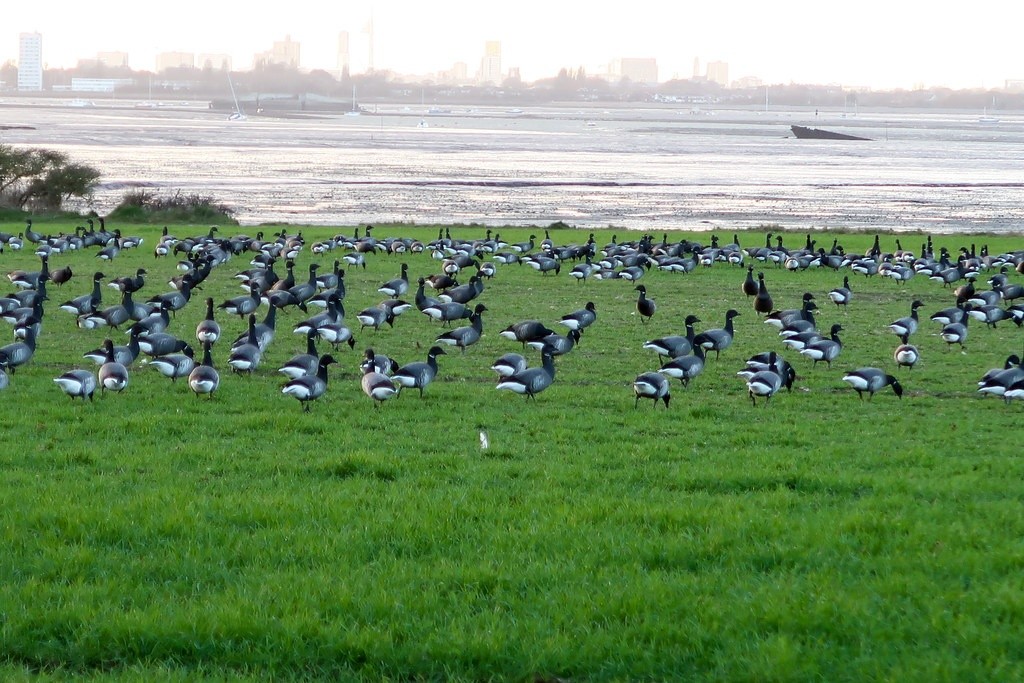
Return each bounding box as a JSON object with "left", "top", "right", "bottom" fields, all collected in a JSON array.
[
  {"left": 979, "top": 106, "right": 1000, "bottom": 124},
  {"left": 790, "top": 125, "right": 872, "bottom": 140}
]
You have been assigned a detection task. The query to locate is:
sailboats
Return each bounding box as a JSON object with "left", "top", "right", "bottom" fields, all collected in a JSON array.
[
  {"left": 344, "top": 84, "right": 361, "bottom": 117},
  {"left": 417, "top": 88, "right": 428, "bottom": 127},
  {"left": 226, "top": 67, "right": 247, "bottom": 122}
]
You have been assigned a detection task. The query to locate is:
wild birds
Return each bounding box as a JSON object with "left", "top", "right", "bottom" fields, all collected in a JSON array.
[{"left": 1, "top": 210, "right": 1022, "bottom": 414}]
